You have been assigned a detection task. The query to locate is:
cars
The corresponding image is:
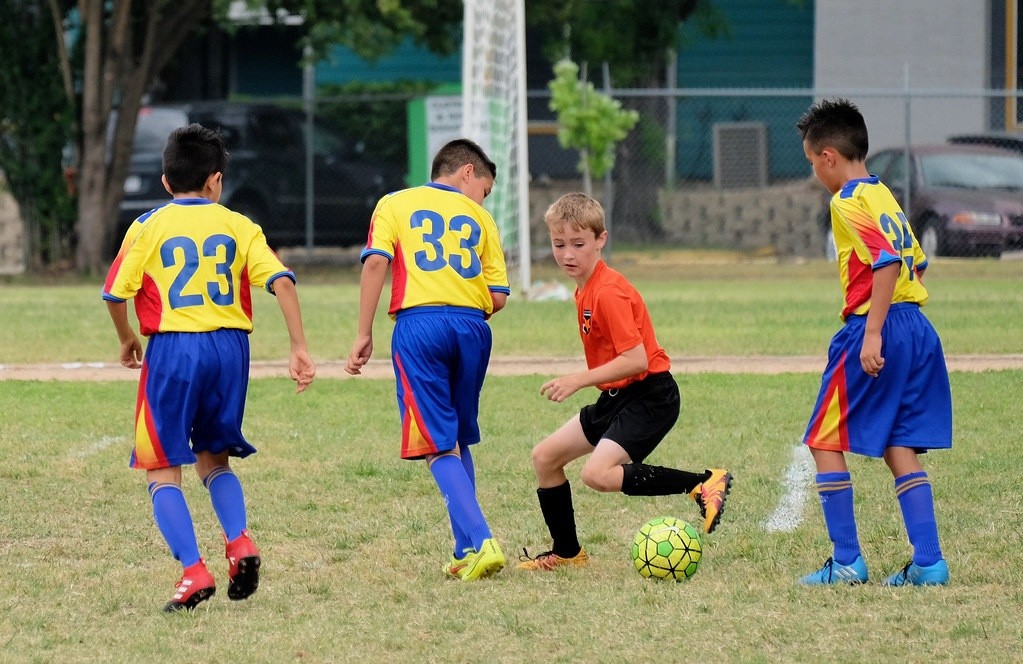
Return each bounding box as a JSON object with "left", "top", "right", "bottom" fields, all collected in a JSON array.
[{"left": 816, "top": 144, "right": 1023, "bottom": 261}]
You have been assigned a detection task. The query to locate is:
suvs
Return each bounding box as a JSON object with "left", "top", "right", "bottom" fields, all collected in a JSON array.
[{"left": 61, "top": 100, "right": 407, "bottom": 263}]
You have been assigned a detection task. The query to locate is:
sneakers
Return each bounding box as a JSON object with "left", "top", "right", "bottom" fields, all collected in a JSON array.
[
  {"left": 163, "top": 558, "right": 217, "bottom": 612},
  {"left": 885, "top": 554, "right": 949, "bottom": 585},
  {"left": 513, "top": 544, "right": 590, "bottom": 572},
  {"left": 443, "top": 552, "right": 478, "bottom": 580},
  {"left": 222, "top": 530, "right": 260, "bottom": 601},
  {"left": 687, "top": 468, "right": 733, "bottom": 533},
  {"left": 800, "top": 556, "right": 870, "bottom": 587},
  {"left": 460, "top": 538, "right": 506, "bottom": 582}
]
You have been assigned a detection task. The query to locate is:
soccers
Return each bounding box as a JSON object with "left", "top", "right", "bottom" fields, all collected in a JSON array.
[{"left": 631, "top": 516, "right": 704, "bottom": 582}]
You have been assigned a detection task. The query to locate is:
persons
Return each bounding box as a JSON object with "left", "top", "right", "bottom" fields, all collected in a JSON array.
[
  {"left": 516, "top": 193, "right": 733, "bottom": 573},
  {"left": 101, "top": 123, "right": 316, "bottom": 613},
  {"left": 797, "top": 99, "right": 954, "bottom": 586},
  {"left": 345, "top": 139, "right": 510, "bottom": 583}
]
